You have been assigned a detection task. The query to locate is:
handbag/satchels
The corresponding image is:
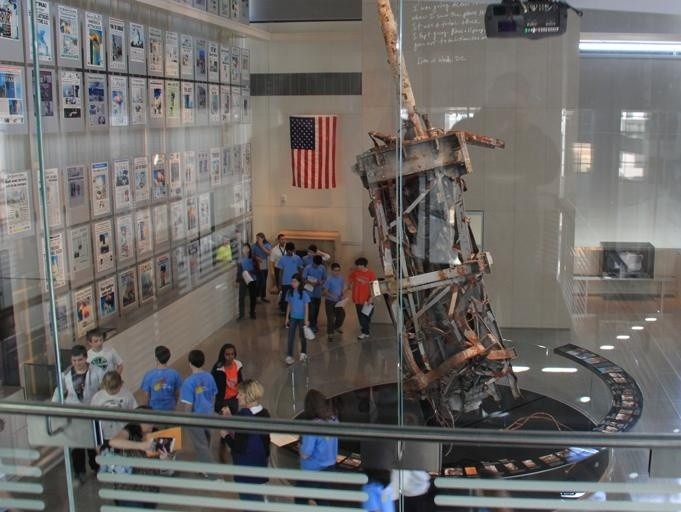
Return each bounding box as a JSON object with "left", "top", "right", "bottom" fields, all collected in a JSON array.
[{"left": 101, "top": 450, "right": 133, "bottom": 474}]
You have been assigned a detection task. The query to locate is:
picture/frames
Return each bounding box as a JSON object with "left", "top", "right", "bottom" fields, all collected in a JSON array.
[{"left": 465, "top": 210, "right": 484, "bottom": 252}]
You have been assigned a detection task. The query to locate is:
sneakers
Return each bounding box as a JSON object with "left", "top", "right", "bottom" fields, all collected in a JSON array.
[
  {"left": 311, "top": 326, "right": 344, "bottom": 340},
  {"left": 358, "top": 333, "right": 368, "bottom": 339},
  {"left": 285, "top": 352, "right": 307, "bottom": 364}
]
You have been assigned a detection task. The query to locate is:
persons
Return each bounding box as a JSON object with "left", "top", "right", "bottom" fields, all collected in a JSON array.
[
  {"left": 50, "top": 329, "right": 183, "bottom": 509},
  {"left": 361, "top": 469, "right": 395, "bottom": 512},
  {"left": 322, "top": 263, "right": 345, "bottom": 341},
  {"left": 220, "top": 380, "right": 271, "bottom": 502},
  {"left": 211, "top": 343, "right": 244, "bottom": 444},
  {"left": 216, "top": 230, "right": 330, "bottom": 365},
  {"left": 391, "top": 412, "right": 431, "bottom": 512},
  {"left": 346, "top": 257, "right": 378, "bottom": 341},
  {"left": 178, "top": 349, "right": 219, "bottom": 478},
  {"left": 294, "top": 389, "right": 338, "bottom": 505}
]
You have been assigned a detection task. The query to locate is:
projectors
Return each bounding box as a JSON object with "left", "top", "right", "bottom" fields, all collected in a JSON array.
[{"left": 484, "top": 0, "right": 584, "bottom": 41}]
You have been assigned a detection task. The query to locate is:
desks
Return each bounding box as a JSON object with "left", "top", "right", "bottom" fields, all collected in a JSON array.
[{"left": 573, "top": 274, "right": 676, "bottom": 315}]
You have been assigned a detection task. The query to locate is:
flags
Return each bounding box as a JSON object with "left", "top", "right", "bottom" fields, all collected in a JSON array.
[{"left": 289, "top": 114, "right": 336, "bottom": 190}]
